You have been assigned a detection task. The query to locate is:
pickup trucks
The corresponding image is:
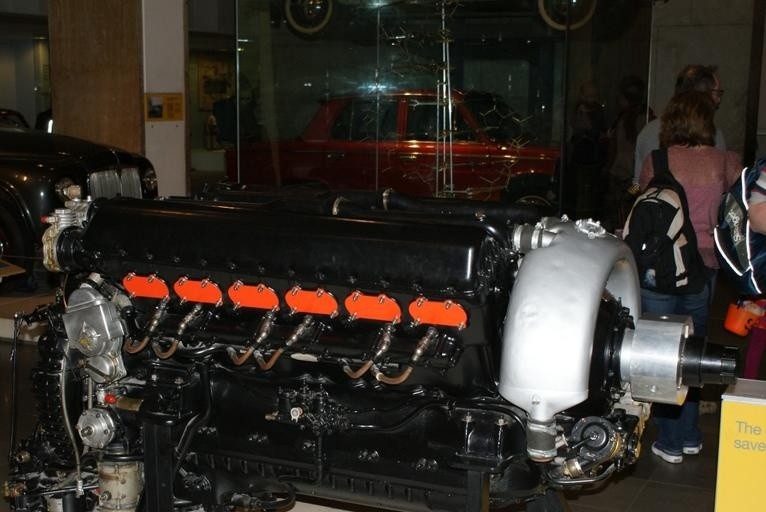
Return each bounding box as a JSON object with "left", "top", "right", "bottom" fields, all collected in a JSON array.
[{"left": 224, "top": 87, "right": 559, "bottom": 214}]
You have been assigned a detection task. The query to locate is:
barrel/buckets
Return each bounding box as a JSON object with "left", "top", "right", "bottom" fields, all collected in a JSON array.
[{"left": 724, "top": 303, "right": 758, "bottom": 337}]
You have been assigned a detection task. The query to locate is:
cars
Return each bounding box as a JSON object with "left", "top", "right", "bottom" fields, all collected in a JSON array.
[{"left": 0, "top": 102, "right": 159, "bottom": 272}]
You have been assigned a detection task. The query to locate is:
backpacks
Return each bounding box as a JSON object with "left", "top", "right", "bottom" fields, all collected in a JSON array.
[
  {"left": 622, "top": 147, "right": 705, "bottom": 297},
  {"left": 713, "top": 158, "right": 766, "bottom": 300}
]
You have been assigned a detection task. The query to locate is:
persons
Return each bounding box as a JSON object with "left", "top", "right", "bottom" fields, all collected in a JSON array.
[
  {"left": 553, "top": 73, "right": 657, "bottom": 234},
  {"left": 713, "top": 148, "right": 766, "bottom": 382},
  {"left": 638, "top": 87, "right": 743, "bottom": 465},
  {"left": 630, "top": 63, "right": 727, "bottom": 183}
]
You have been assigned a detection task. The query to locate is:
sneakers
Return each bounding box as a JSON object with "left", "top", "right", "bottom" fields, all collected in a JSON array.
[{"left": 651, "top": 440, "right": 703, "bottom": 464}]
